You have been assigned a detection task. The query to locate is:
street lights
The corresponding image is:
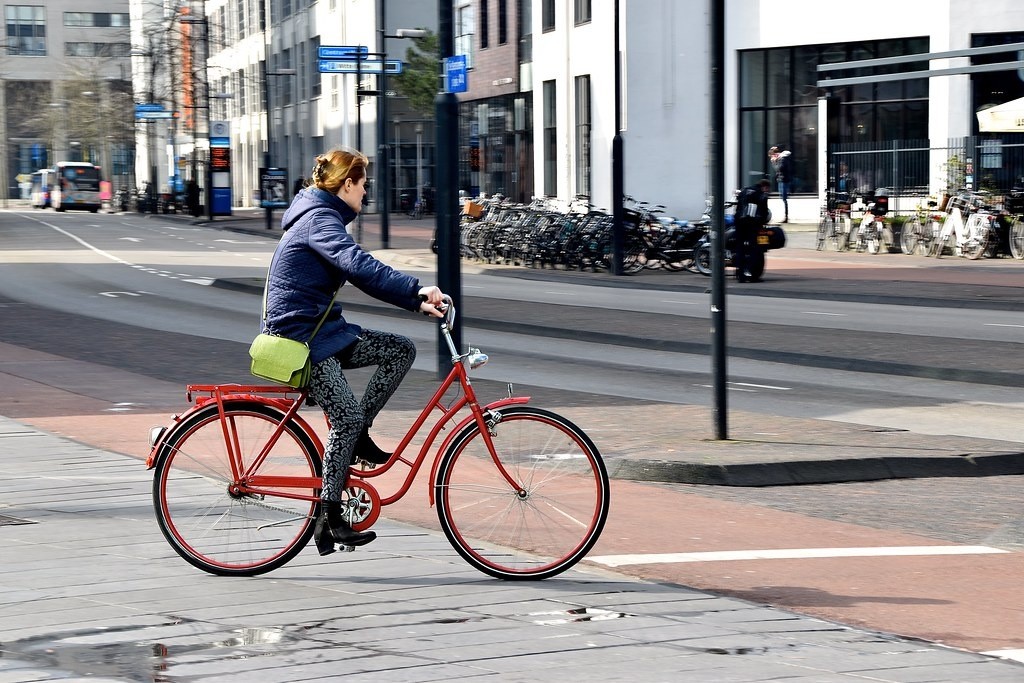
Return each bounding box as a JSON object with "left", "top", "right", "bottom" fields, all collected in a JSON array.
[{"left": 178, "top": 14, "right": 214, "bottom": 221}]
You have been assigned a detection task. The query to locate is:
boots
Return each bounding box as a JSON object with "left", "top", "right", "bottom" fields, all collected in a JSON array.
[
  {"left": 314, "top": 500, "right": 377, "bottom": 556},
  {"left": 347, "top": 425, "right": 393, "bottom": 465}
]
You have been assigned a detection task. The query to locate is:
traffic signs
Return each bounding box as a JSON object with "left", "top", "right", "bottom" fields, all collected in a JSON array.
[{"left": 317, "top": 58, "right": 402, "bottom": 74}]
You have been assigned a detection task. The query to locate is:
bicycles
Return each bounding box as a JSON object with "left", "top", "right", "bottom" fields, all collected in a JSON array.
[
  {"left": 410, "top": 195, "right": 427, "bottom": 217},
  {"left": 112, "top": 187, "right": 195, "bottom": 216},
  {"left": 814, "top": 180, "right": 1023, "bottom": 261},
  {"left": 143, "top": 285, "right": 611, "bottom": 587},
  {"left": 428, "top": 189, "right": 785, "bottom": 280}
]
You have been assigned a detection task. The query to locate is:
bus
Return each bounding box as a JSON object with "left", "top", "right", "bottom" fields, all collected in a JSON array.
[
  {"left": 50, "top": 161, "right": 102, "bottom": 213},
  {"left": 27, "top": 169, "right": 55, "bottom": 209}
]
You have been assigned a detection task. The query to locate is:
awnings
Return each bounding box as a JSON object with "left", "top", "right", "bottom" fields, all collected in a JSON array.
[{"left": 976, "top": 96, "right": 1024, "bottom": 132}]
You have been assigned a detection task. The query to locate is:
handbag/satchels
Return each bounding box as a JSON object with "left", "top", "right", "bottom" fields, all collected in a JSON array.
[{"left": 248, "top": 334, "right": 311, "bottom": 388}]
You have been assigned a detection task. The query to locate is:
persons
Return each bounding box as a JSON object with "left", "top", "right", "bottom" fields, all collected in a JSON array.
[
  {"left": 261, "top": 151, "right": 447, "bottom": 556},
  {"left": 734, "top": 180, "right": 770, "bottom": 283},
  {"left": 768, "top": 144, "right": 792, "bottom": 223},
  {"left": 294, "top": 179, "right": 306, "bottom": 196}
]
